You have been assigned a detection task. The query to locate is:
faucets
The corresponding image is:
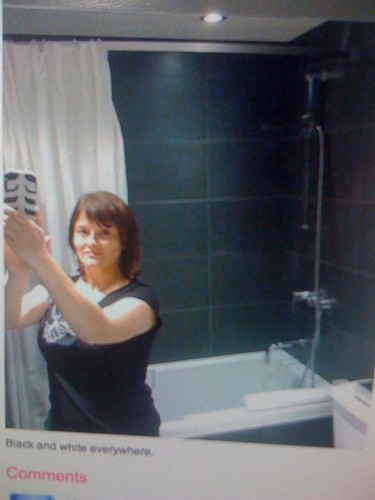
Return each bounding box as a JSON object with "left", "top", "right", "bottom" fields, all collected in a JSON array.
[{"left": 291, "top": 291, "right": 320, "bottom": 310}]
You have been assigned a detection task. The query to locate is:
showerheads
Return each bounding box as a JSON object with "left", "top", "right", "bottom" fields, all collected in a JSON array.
[{"left": 303, "top": 113, "right": 323, "bottom": 134}]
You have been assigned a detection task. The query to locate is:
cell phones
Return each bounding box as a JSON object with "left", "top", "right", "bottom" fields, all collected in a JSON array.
[{"left": 3, "top": 170, "right": 39, "bottom": 222}]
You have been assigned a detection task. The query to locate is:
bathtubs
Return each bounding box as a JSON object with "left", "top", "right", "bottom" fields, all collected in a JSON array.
[{"left": 143, "top": 345, "right": 338, "bottom": 448}]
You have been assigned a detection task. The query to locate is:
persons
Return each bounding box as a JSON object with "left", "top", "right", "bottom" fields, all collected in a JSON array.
[{"left": 2, "top": 189, "right": 164, "bottom": 437}]
